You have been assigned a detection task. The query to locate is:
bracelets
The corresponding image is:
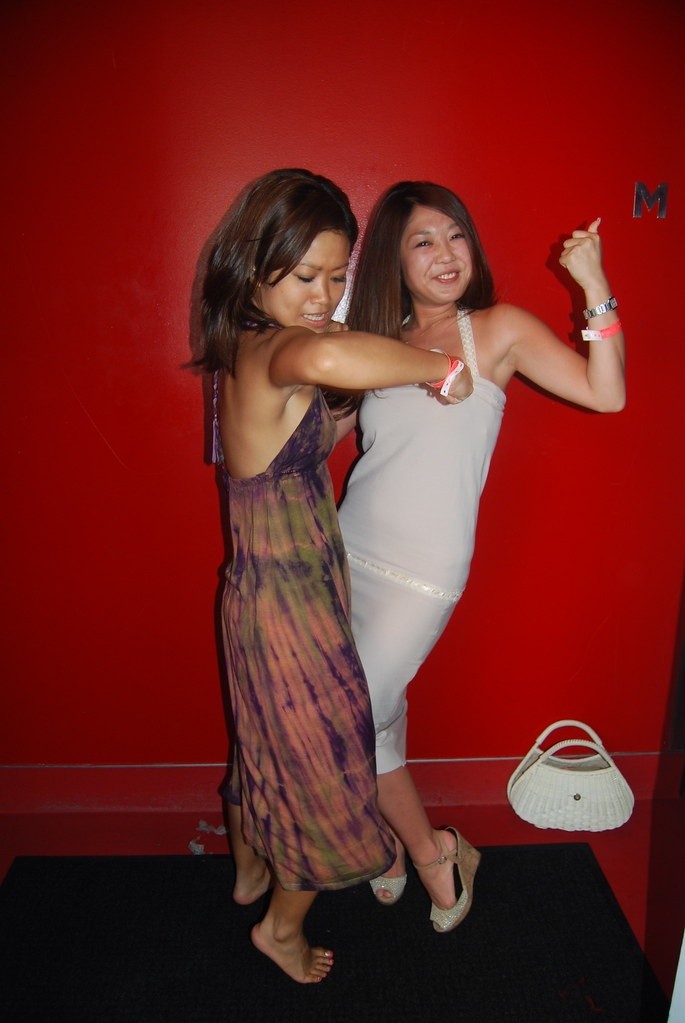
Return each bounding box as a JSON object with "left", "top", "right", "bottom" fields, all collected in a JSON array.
[
  {"left": 581, "top": 320, "right": 623, "bottom": 343},
  {"left": 425, "top": 348, "right": 464, "bottom": 398},
  {"left": 583, "top": 296, "right": 618, "bottom": 321}
]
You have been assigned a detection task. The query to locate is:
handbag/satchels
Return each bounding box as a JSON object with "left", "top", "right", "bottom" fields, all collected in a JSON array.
[{"left": 507, "top": 720, "right": 634, "bottom": 833}]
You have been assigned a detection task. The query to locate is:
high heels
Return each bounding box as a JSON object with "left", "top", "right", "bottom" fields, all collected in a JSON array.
[
  {"left": 413, "top": 826, "right": 482, "bottom": 932},
  {"left": 369, "top": 874, "right": 407, "bottom": 904}
]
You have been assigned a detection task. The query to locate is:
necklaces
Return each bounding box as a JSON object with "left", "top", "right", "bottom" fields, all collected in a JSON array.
[{"left": 405, "top": 314, "right": 456, "bottom": 344}]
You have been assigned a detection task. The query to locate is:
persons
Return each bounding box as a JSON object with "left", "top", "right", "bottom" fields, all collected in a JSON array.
[
  {"left": 322, "top": 179, "right": 626, "bottom": 935},
  {"left": 199, "top": 167, "right": 474, "bottom": 985}
]
